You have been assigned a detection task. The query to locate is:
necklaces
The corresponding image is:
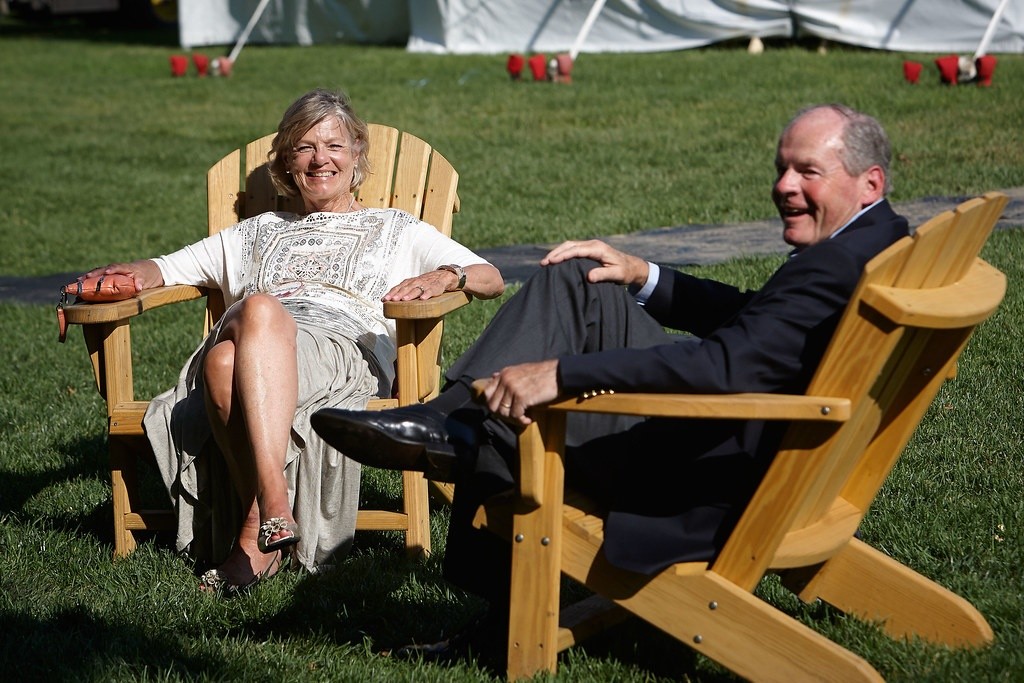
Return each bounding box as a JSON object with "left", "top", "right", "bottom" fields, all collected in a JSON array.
[{"left": 345, "top": 194, "right": 357, "bottom": 215}]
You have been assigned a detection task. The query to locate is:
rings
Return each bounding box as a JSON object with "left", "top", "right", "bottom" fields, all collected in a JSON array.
[
  {"left": 415, "top": 286, "right": 424, "bottom": 294},
  {"left": 500, "top": 400, "right": 511, "bottom": 409}
]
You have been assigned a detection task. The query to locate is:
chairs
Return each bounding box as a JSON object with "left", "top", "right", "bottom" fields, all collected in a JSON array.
[
  {"left": 63, "top": 122, "right": 474, "bottom": 556},
  {"left": 468, "top": 191, "right": 1012, "bottom": 683}
]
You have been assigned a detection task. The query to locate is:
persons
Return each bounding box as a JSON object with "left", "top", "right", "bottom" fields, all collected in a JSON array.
[
  {"left": 309, "top": 104, "right": 912, "bottom": 666},
  {"left": 76, "top": 89, "right": 505, "bottom": 597}
]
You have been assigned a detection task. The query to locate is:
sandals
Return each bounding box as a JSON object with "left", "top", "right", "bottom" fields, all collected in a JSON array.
[
  {"left": 259, "top": 509, "right": 300, "bottom": 552},
  {"left": 200, "top": 556, "right": 280, "bottom": 595}
]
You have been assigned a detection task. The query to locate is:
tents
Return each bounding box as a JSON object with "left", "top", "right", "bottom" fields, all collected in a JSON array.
[{"left": 178, "top": 0, "right": 1024, "bottom": 85}]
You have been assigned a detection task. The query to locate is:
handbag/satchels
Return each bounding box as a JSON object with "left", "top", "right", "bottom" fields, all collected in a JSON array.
[{"left": 57, "top": 270, "right": 136, "bottom": 346}]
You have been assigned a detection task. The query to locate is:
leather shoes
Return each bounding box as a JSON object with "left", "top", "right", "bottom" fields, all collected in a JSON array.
[
  {"left": 394, "top": 620, "right": 509, "bottom": 668},
  {"left": 312, "top": 402, "right": 460, "bottom": 482}
]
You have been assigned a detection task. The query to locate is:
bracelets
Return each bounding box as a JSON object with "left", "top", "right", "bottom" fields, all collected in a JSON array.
[{"left": 438, "top": 263, "right": 467, "bottom": 292}]
